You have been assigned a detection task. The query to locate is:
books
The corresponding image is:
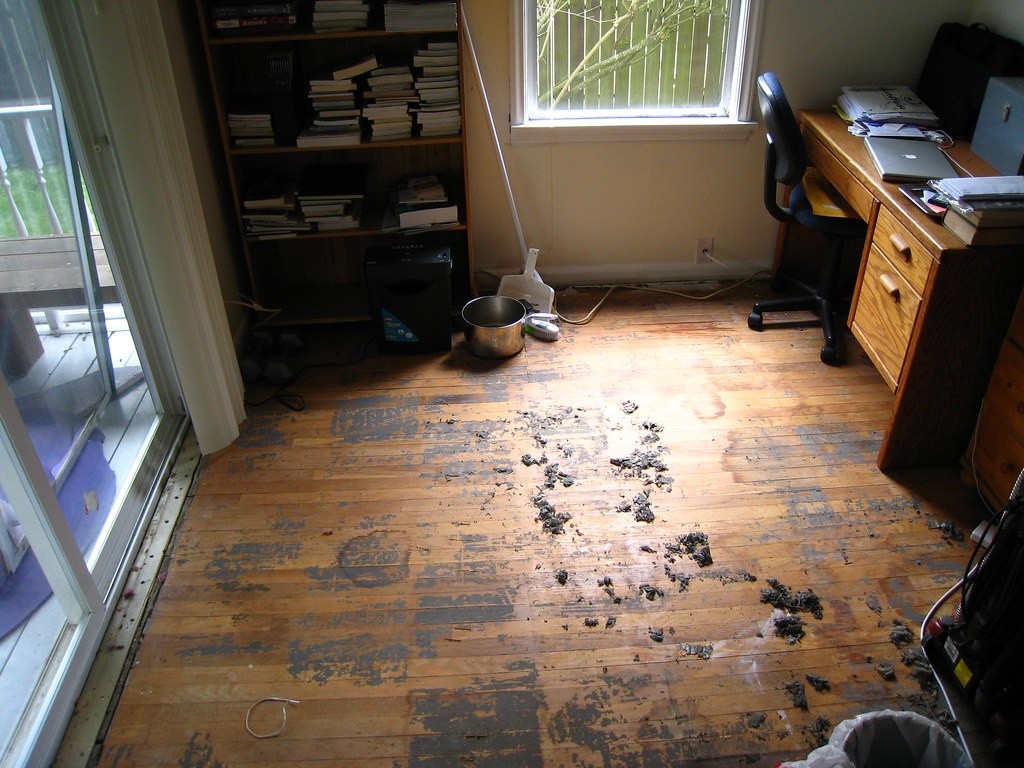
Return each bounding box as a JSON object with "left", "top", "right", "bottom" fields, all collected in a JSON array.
[
  {"left": 227, "top": 42, "right": 464, "bottom": 149},
  {"left": 211, "top": 0, "right": 300, "bottom": 32},
  {"left": 312, "top": 0, "right": 369, "bottom": 34},
  {"left": 838, "top": 84, "right": 1024, "bottom": 245},
  {"left": 383, "top": 0, "right": 455, "bottom": 31},
  {"left": 242, "top": 174, "right": 460, "bottom": 241}
]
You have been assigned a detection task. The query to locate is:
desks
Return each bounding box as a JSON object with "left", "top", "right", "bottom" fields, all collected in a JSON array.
[{"left": 772, "top": 109, "right": 1024, "bottom": 473}]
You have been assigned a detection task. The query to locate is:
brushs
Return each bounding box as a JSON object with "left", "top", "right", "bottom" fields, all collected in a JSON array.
[{"left": 524, "top": 313, "right": 560, "bottom": 341}]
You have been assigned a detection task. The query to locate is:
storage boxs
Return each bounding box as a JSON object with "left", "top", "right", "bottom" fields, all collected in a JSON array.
[{"left": 971, "top": 76, "right": 1024, "bottom": 177}]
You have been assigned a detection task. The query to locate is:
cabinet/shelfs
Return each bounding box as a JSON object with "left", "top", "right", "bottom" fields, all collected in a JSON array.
[
  {"left": 959, "top": 287, "right": 1024, "bottom": 512},
  {"left": 194, "top": 0, "right": 479, "bottom": 333}
]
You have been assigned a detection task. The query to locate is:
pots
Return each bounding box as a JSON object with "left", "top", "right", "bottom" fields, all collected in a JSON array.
[{"left": 455, "top": 295, "right": 533, "bottom": 358}]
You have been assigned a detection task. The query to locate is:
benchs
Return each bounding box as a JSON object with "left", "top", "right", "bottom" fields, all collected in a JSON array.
[{"left": 0, "top": 234, "right": 122, "bottom": 378}]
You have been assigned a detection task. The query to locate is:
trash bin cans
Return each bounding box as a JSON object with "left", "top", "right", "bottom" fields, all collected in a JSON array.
[{"left": 842, "top": 717, "right": 975, "bottom": 768}]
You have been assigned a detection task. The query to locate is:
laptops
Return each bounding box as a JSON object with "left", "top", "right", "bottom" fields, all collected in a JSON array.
[{"left": 865, "top": 137, "right": 959, "bottom": 182}]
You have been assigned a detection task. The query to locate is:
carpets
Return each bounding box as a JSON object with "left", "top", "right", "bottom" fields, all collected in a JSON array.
[{"left": 0, "top": 407, "right": 117, "bottom": 642}]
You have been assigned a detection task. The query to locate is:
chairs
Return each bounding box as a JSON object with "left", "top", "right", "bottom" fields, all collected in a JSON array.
[{"left": 748, "top": 71, "right": 869, "bottom": 365}]
